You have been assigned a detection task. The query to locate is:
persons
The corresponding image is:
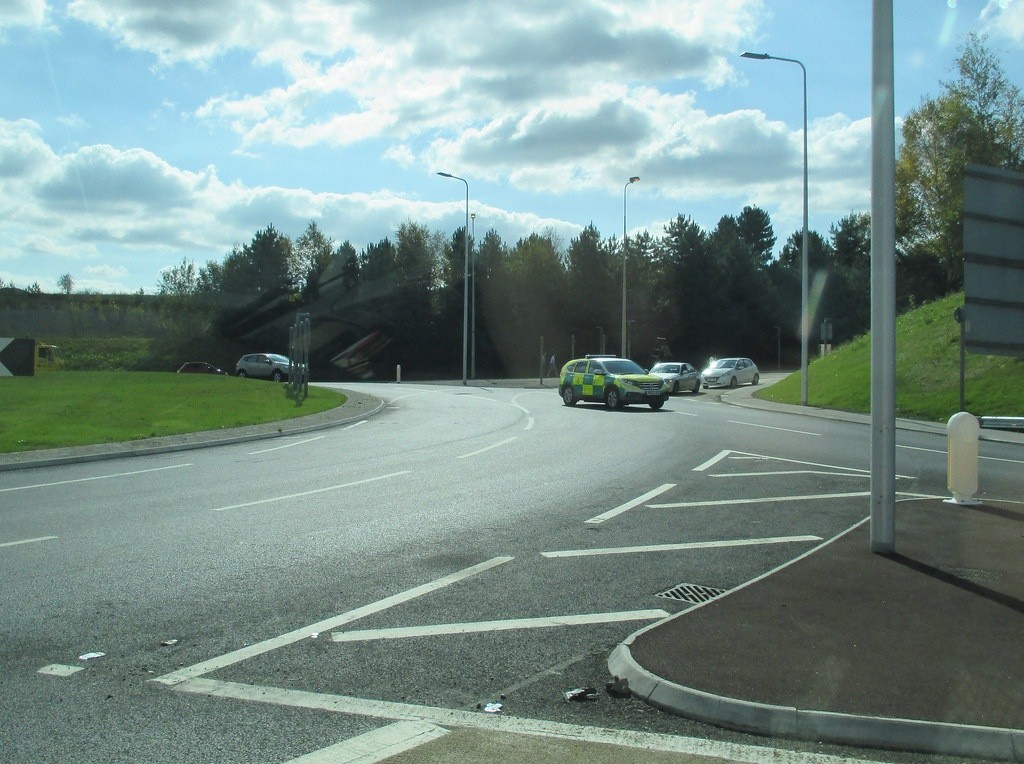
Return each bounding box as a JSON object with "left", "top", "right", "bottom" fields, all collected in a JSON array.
[{"left": 539, "top": 352, "right": 559, "bottom": 378}]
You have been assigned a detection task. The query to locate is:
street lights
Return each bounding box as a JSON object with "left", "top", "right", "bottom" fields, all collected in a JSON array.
[
  {"left": 436, "top": 171, "right": 468, "bottom": 384},
  {"left": 470, "top": 213, "right": 478, "bottom": 380},
  {"left": 621, "top": 176, "right": 641, "bottom": 363},
  {"left": 740, "top": 50, "right": 809, "bottom": 408}
]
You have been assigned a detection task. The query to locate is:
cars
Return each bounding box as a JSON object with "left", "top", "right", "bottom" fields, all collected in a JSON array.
[
  {"left": 177, "top": 361, "right": 230, "bottom": 376},
  {"left": 700, "top": 357, "right": 760, "bottom": 388},
  {"left": 236, "top": 353, "right": 309, "bottom": 383},
  {"left": 646, "top": 362, "right": 702, "bottom": 395},
  {"left": 558, "top": 354, "right": 670, "bottom": 412}
]
